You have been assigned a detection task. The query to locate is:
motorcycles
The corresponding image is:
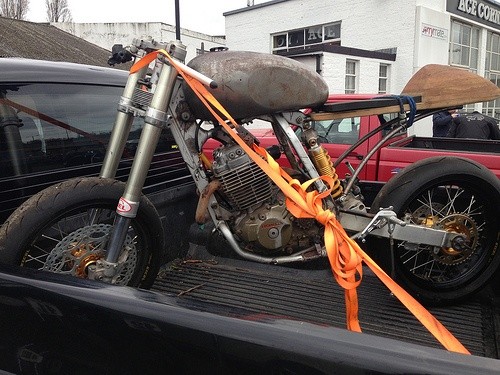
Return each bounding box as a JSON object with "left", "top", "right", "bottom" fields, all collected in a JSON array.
[{"left": 0, "top": 37, "right": 499, "bottom": 304}]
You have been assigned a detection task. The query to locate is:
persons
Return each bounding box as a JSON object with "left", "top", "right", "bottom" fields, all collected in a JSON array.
[
  {"left": 447, "top": 111, "right": 500, "bottom": 139},
  {"left": 433, "top": 108, "right": 456, "bottom": 138}
]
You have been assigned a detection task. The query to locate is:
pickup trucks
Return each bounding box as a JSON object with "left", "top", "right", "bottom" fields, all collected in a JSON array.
[
  {"left": 201, "top": 94, "right": 499, "bottom": 186},
  {"left": 0, "top": 56, "right": 498, "bottom": 375}
]
